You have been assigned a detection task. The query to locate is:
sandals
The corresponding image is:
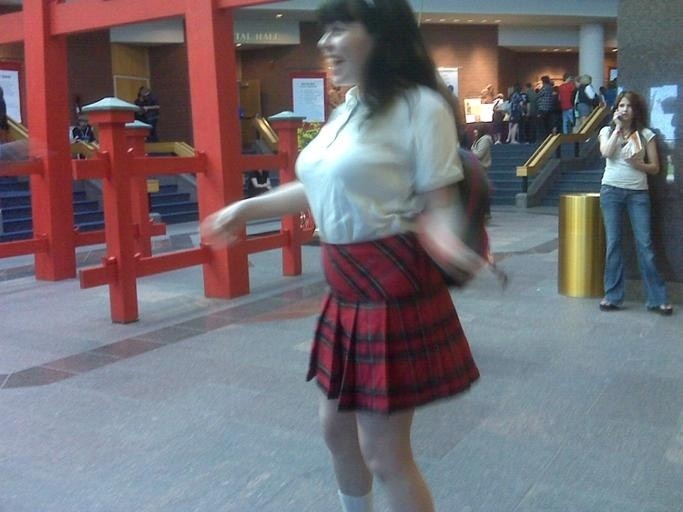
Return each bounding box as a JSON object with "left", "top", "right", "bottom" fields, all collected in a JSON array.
[{"left": 599, "top": 298, "right": 672, "bottom": 314}]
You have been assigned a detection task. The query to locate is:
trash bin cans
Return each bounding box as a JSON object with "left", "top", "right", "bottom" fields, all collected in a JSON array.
[{"left": 559, "top": 194, "right": 603, "bottom": 298}]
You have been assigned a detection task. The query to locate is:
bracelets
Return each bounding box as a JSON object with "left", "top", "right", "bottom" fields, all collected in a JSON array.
[
  {"left": 611, "top": 129, "right": 622, "bottom": 136},
  {"left": 639, "top": 160, "right": 643, "bottom": 170}
]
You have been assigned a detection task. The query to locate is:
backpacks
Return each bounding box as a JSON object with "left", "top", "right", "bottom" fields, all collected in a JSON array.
[{"left": 444, "top": 152, "right": 491, "bottom": 284}]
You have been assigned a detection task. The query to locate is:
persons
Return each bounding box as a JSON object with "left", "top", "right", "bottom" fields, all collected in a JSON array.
[
  {"left": 0, "top": 87, "right": 9, "bottom": 144},
  {"left": 493, "top": 74, "right": 617, "bottom": 146},
  {"left": 469, "top": 126, "right": 493, "bottom": 220},
  {"left": 73, "top": 115, "right": 94, "bottom": 160},
  {"left": 597, "top": 91, "right": 672, "bottom": 316},
  {"left": 198, "top": 1, "right": 502, "bottom": 510},
  {"left": 247, "top": 169, "right": 271, "bottom": 197},
  {"left": 480, "top": 84, "right": 494, "bottom": 104},
  {"left": 134, "top": 86, "right": 159, "bottom": 142}
]
